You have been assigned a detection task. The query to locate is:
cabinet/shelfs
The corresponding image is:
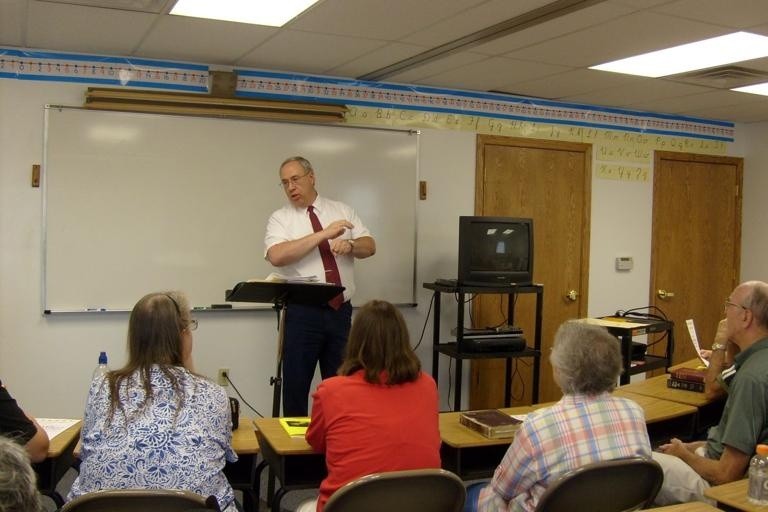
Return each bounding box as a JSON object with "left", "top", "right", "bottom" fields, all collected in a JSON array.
[
  {"left": 588, "top": 314, "right": 676, "bottom": 385},
  {"left": 422, "top": 280, "right": 544, "bottom": 411}
]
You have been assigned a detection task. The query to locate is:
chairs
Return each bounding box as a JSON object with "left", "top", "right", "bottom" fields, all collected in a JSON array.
[
  {"left": 322, "top": 468, "right": 466, "bottom": 511},
  {"left": 531, "top": 456, "right": 661, "bottom": 510},
  {"left": 59, "top": 489, "right": 219, "bottom": 512}
]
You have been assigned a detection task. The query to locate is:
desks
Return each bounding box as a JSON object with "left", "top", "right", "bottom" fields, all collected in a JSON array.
[
  {"left": 249, "top": 415, "right": 319, "bottom": 506},
  {"left": 73, "top": 417, "right": 260, "bottom": 506},
  {"left": 23, "top": 417, "right": 80, "bottom": 489},
  {"left": 516, "top": 392, "right": 688, "bottom": 429},
  {"left": 646, "top": 502, "right": 715, "bottom": 512},
  {"left": 632, "top": 375, "right": 719, "bottom": 407},
  {"left": 707, "top": 470, "right": 768, "bottom": 510},
  {"left": 671, "top": 357, "right": 709, "bottom": 375},
  {"left": 438, "top": 403, "right": 537, "bottom": 471}
]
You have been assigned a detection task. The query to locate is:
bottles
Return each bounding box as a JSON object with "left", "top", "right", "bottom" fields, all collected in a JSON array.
[
  {"left": 748, "top": 444, "right": 768, "bottom": 508},
  {"left": 94, "top": 351, "right": 109, "bottom": 384}
]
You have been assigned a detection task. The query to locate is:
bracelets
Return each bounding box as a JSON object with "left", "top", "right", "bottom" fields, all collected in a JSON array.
[{"left": 344, "top": 239, "right": 354, "bottom": 253}]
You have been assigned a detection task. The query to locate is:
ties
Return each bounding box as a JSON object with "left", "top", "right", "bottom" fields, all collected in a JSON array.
[{"left": 306, "top": 205, "right": 344, "bottom": 312}]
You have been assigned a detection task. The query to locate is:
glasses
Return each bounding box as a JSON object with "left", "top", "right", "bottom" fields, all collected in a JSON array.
[
  {"left": 184, "top": 319, "right": 198, "bottom": 332},
  {"left": 278, "top": 171, "right": 310, "bottom": 189}
]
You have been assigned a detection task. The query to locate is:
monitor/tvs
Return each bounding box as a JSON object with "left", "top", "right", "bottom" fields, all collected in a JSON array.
[{"left": 458, "top": 216, "right": 533, "bottom": 288}]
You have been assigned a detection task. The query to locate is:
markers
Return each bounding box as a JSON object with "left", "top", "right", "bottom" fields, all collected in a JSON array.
[
  {"left": 85, "top": 308, "right": 106, "bottom": 312},
  {"left": 191, "top": 307, "right": 209, "bottom": 309}
]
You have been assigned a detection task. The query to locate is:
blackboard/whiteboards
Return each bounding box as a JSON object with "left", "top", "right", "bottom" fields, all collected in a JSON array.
[{"left": 40, "top": 104, "right": 421, "bottom": 314}]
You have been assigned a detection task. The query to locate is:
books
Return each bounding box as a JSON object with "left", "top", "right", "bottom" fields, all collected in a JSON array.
[
  {"left": 461, "top": 409, "right": 523, "bottom": 438},
  {"left": 264, "top": 272, "right": 319, "bottom": 284},
  {"left": 281, "top": 416, "right": 311, "bottom": 438},
  {"left": 667, "top": 368, "right": 707, "bottom": 392}
]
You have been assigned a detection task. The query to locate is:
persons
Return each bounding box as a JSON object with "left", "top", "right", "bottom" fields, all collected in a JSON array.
[
  {"left": 701, "top": 319, "right": 737, "bottom": 400},
  {"left": 465, "top": 320, "right": 653, "bottom": 512},
  {"left": 280, "top": 300, "right": 440, "bottom": 512},
  {"left": 1, "top": 382, "right": 58, "bottom": 512},
  {"left": 1, "top": 434, "right": 42, "bottom": 512},
  {"left": 264, "top": 156, "right": 375, "bottom": 415},
  {"left": 650, "top": 280, "right": 767, "bottom": 512},
  {"left": 65, "top": 292, "right": 239, "bottom": 512}
]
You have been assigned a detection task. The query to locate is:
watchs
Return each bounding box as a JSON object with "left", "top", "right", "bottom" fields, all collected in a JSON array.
[{"left": 711, "top": 343, "right": 727, "bottom": 350}]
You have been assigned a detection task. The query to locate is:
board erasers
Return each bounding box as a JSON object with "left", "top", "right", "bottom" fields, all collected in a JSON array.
[{"left": 210, "top": 304, "right": 232, "bottom": 309}]
